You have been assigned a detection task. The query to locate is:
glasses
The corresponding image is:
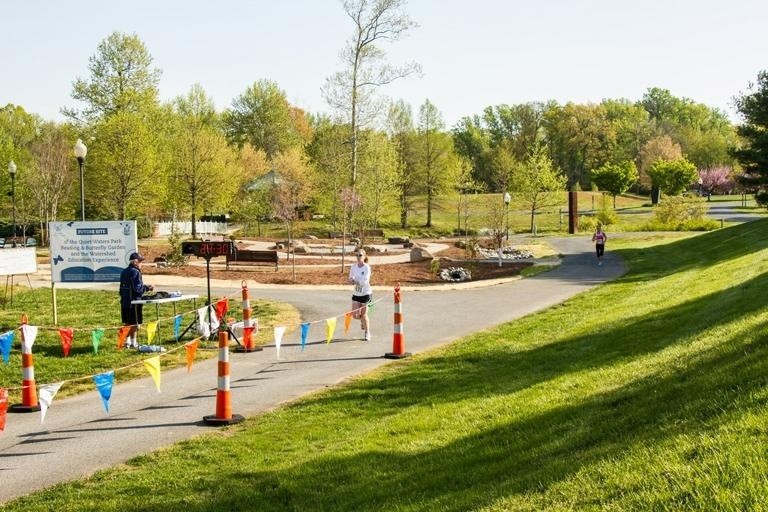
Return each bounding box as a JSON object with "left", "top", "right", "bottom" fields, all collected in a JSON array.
[{"left": 355, "top": 254, "right": 363, "bottom": 257}]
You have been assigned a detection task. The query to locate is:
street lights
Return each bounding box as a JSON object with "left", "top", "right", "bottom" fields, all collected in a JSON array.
[
  {"left": 8, "top": 161, "right": 17, "bottom": 247},
  {"left": 504, "top": 192, "right": 510, "bottom": 241},
  {"left": 74, "top": 139, "right": 88, "bottom": 222},
  {"left": 697, "top": 178, "right": 702, "bottom": 194}
]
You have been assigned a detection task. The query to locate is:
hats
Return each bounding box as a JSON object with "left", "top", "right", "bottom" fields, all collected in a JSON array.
[{"left": 129, "top": 252, "right": 145, "bottom": 261}]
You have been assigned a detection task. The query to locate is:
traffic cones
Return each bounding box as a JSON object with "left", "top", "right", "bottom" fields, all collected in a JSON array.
[
  {"left": 234, "top": 287, "right": 263, "bottom": 352},
  {"left": 202, "top": 332, "right": 244, "bottom": 426},
  {"left": 385, "top": 291, "right": 411, "bottom": 359},
  {"left": 7, "top": 327, "right": 41, "bottom": 412}
]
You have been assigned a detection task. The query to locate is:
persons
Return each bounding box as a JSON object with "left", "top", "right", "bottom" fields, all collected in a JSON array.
[
  {"left": 592, "top": 225, "right": 607, "bottom": 266},
  {"left": 343, "top": 249, "right": 372, "bottom": 341},
  {"left": 120, "top": 253, "right": 153, "bottom": 348}
]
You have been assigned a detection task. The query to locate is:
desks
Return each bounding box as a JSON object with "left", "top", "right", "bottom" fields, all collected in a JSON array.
[{"left": 128, "top": 292, "right": 200, "bottom": 347}]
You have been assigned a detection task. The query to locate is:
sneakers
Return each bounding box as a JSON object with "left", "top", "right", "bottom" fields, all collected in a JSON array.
[
  {"left": 361, "top": 319, "right": 371, "bottom": 341},
  {"left": 125, "top": 343, "right": 140, "bottom": 349}
]
